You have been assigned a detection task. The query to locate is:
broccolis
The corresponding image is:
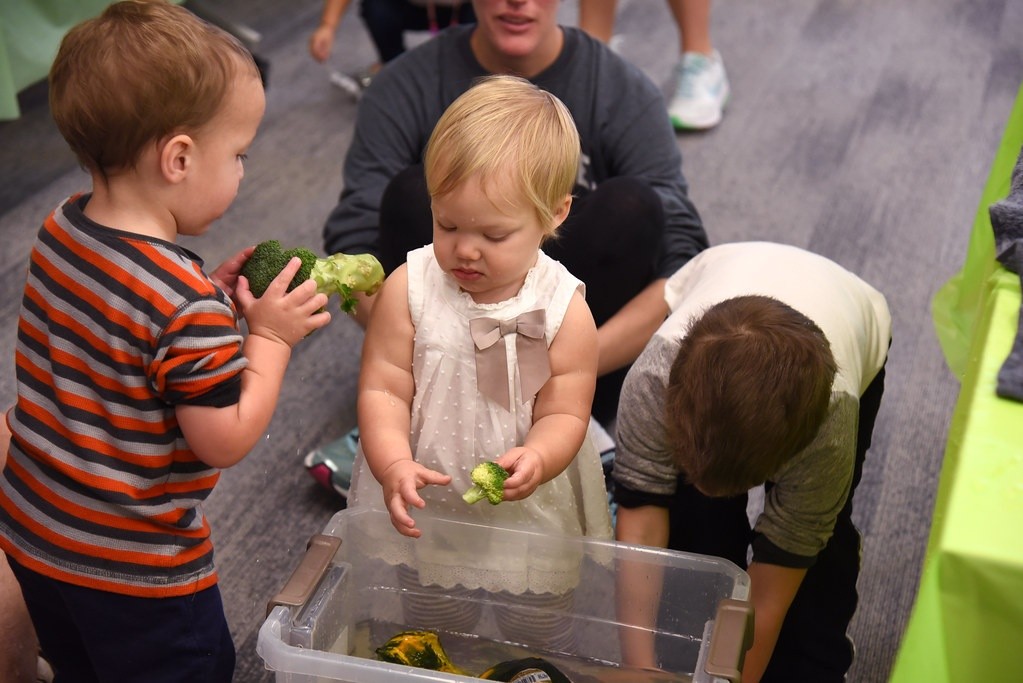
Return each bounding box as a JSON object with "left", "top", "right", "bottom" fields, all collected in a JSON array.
[
  {"left": 462, "top": 462, "right": 509, "bottom": 506},
  {"left": 242, "top": 240, "right": 386, "bottom": 335}
]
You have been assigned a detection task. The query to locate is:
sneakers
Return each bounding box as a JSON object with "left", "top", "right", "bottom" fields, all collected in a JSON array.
[
  {"left": 666, "top": 47, "right": 730, "bottom": 129},
  {"left": 596, "top": 446, "right": 617, "bottom": 535},
  {"left": 303, "top": 428, "right": 361, "bottom": 507}
]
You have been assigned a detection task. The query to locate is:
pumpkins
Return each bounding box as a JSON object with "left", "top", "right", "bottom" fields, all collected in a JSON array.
[{"left": 477, "top": 655, "right": 574, "bottom": 683}]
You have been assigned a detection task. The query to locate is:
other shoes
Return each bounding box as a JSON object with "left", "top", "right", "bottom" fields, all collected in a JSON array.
[{"left": 328, "top": 62, "right": 385, "bottom": 105}]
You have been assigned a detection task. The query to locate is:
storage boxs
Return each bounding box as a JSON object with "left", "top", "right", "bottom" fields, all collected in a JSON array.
[{"left": 259, "top": 503, "right": 750, "bottom": 683}]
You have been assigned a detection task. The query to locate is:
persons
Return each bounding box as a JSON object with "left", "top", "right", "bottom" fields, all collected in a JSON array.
[
  {"left": 345, "top": 76, "right": 615, "bottom": 657},
  {"left": 310, "top": 1, "right": 478, "bottom": 104},
  {"left": 1, "top": 9, "right": 332, "bottom": 683},
  {"left": 321, "top": 1, "right": 710, "bottom": 438},
  {"left": 577, "top": 1, "right": 729, "bottom": 131},
  {"left": 615, "top": 240, "right": 891, "bottom": 683}
]
